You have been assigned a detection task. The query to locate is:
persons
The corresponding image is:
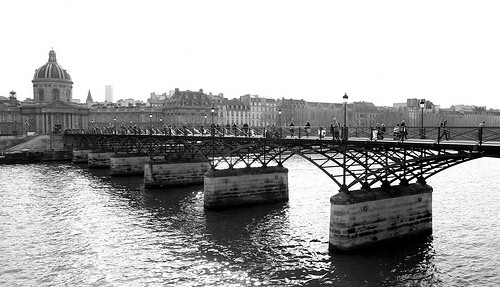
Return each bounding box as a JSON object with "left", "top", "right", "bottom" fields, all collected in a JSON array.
[
  {"left": 478, "top": 121, "right": 485, "bottom": 131},
  {"left": 375, "top": 122, "right": 386, "bottom": 140},
  {"left": 393, "top": 120, "right": 408, "bottom": 141},
  {"left": 304, "top": 122, "right": 310, "bottom": 138},
  {"left": 290, "top": 122, "right": 294, "bottom": 137},
  {"left": 330, "top": 120, "right": 340, "bottom": 140}
]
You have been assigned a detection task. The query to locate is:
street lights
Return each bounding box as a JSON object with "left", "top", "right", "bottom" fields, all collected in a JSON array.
[
  {"left": 419, "top": 99, "right": 426, "bottom": 139},
  {"left": 276, "top": 107, "right": 283, "bottom": 133},
  {"left": 204, "top": 114, "right": 208, "bottom": 127},
  {"left": 211, "top": 107, "right": 215, "bottom": 124},
  {"left": 91, "top": 119, "right": 94, "bottom": 127},
  {"left": 113, "top": 117, "right": 117, "bottom": 133},
  {"left": 341, "top": 92, "right": 349, "bottom": 141},
  {"left": 149, "top": 113, "right": 153, "bottom": 130}
]
